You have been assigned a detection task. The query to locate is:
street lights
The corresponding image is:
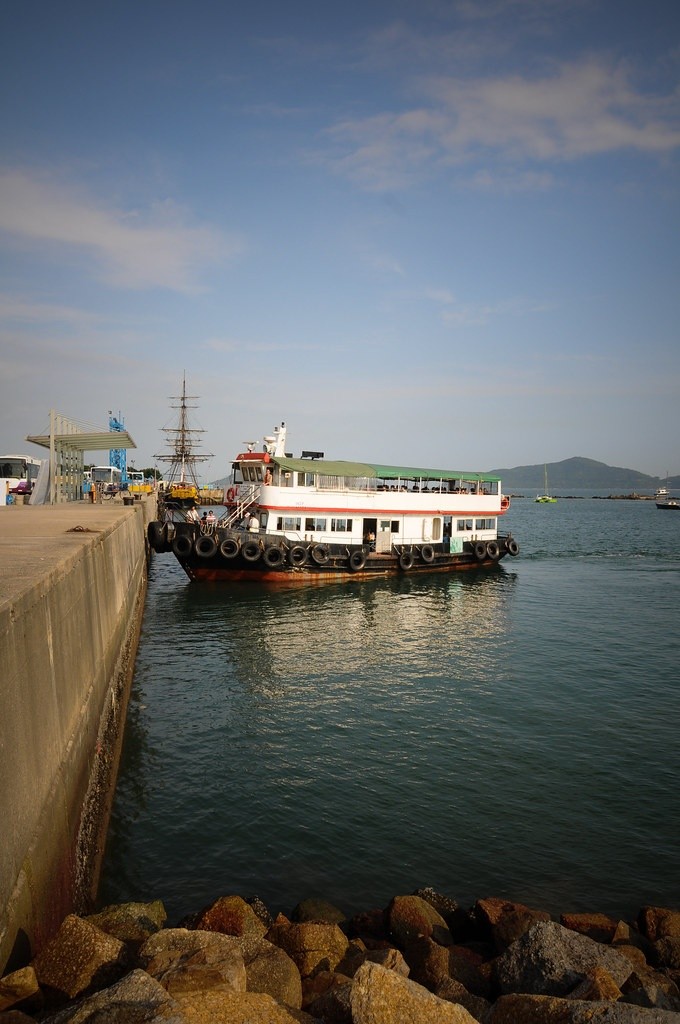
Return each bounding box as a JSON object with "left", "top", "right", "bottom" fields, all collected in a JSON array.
[{"left": 130, "top": 459, "right": 135, "bottom": 468}]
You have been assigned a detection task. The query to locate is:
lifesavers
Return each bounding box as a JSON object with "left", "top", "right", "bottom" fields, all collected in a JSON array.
[
  {"left": 172, "top": 534, "right": 193, "bottom": 555},
  {"left": 241, "top": 541, "right": 261, "bottom": 561},
  {"left": 506, "top": 541, "right": 519, "bottom": 556},
  {"left": 501, "top": 498, "right": 508, "bottom": 508},
  {"left": 418, "top": 545, "right": 434, "bottom": 562},
  {"left": 348, "top": 550, "right": 366, "bottom": 571},
  {"left": 227, "top": 488, "right": 234, "bottom": 501},
  {"left": 219, "top": 538, "right": 241, "bottom": 560},
  {"left": 287, "top": 545, "right": 308, "bottom": 567},
  {"left": 194, "top": 536, "right": 217, "bottom": 559},
  {"left": 309, "top": 543, "right": 330, "bottom": 565},
  {"left": 263, "top": 546, "right": 285, "bottom": 568},
  {"left": 147, "top": 521, "right": 166, "bottom": 553},
  {"left": 397, "top": 552, "right": 414, "bottom": 570},
  {"left": 472, "top": 542, "right": 500, "bottom": 560}
]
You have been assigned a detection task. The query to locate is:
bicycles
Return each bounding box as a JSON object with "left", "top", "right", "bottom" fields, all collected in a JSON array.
[{"left": 100, "top": 482, "right": 131, "bottom": 500}]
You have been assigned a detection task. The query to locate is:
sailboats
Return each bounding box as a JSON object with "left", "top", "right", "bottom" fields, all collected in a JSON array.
[
  {"left": 654, "top": 471, "right": 680, "bottom": 511},
  {"left": 535, "top": 462, "right": 557, "bottom": 503},
  {"left": 150, "top": 368, "right": 217, "bottom": 510}
]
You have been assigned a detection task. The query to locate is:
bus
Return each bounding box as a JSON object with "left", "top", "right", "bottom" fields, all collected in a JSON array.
[
  {"left": 0, "top": 454, "right": 42, "bottom": 495},
  {"left": 55, "top": 465, "right": 144, "bottom": 494}
]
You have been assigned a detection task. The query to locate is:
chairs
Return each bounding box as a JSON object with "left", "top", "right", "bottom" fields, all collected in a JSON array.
[{"left": 377, "top": 485, "right": 475, "bottom": 495}]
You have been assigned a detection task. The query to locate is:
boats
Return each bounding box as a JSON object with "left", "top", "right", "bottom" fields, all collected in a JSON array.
[{"left": 146, "top": 422, "right": 521, "bottom": 584}]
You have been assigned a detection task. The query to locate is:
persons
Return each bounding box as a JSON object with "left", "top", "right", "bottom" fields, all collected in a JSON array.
[
  {"left": 207, "top": 510, "right": 216, "bottom": 523},
  {"left": 366, "top": 531, "right": 376, "bottom": 540},
  {"left": 118, "top": 481, "right": 129, "bottom": 492},
  {"left": 262, "top": 469, "right": 273, "bottom": 485},
  {"left": 375, "top": 483, "right": 492, "bottom": 495},
  {"left": 200, "top": 512, "right": 208, "bottom": 524},
  {"left": 277, "top": 520, "right": 345, "bottom": 531},
  {"left": 249, "top": 511, "right": 260, "bottom": 533},
  {"left": 442, "top": 522, "right": 452, "bottom": 551},
  {"left": 82, "top": 474, "right": 91, "bottom": 494},
  {"left": 187, "top": 505, "right": 202, "bottom": 525}
]
